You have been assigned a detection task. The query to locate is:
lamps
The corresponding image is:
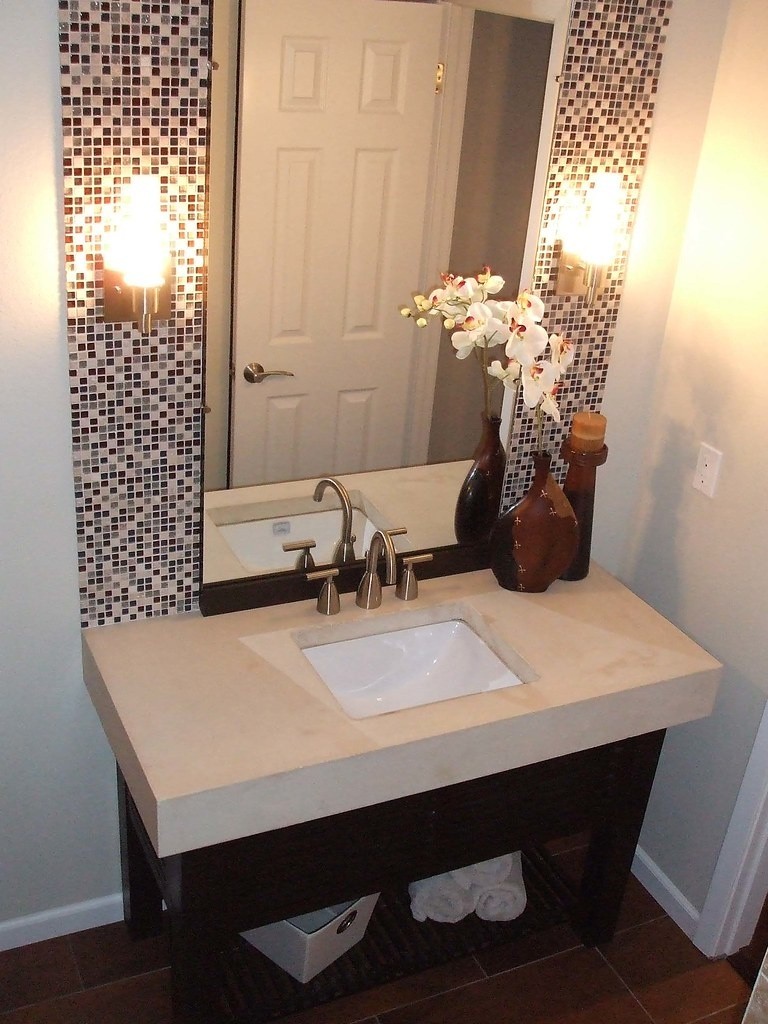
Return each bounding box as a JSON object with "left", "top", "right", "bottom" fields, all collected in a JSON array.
[
  {"left": 546, "top": 171, "right": 630, "bottom": 300},
  {"left": 100, "top": 187, "right": 175, "bottom": 333}
]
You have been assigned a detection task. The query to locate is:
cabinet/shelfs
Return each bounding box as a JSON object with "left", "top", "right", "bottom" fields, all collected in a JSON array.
[{"left": 114, "top": 727, "right": 667, "bottom": 1024}]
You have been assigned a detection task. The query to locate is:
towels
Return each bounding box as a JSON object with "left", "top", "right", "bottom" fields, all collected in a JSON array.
[{"left": 407, "top": 849, "right": 528, "bottom": 924}]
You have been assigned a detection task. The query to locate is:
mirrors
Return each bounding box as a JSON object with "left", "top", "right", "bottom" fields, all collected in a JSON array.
[{"left": 227, "top": 0, "right": 555, "bottom": 487}]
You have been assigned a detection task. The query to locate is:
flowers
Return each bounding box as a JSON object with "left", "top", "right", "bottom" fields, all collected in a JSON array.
[
  {"left": 446, "top": 281, "right": 576, "bottom": 454},
  {"left": 400, "top": 264, "right": 531, "bottom": 418}
]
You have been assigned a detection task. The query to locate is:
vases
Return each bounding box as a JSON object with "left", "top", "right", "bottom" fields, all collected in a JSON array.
[
  {"left": 453, "top": 411, "right": 507, "bottom": 544},
  {"left": 491, "top": 451, "right": 580, "bottom": 594}
]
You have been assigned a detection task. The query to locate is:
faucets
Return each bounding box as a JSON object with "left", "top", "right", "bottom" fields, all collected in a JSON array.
[
  {"left": 355, "top": 529, "right": 397, "bottom": 610},
  {"left": 312, "top": 477, "right": 357, "bottom": 568}
]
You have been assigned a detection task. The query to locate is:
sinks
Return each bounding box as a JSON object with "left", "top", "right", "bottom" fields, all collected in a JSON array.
[
  {"left": 298, "top": 617, "right": 526, "bottom": 722},
  {"left": 214, "top": 506, "right": 399, "bottom": 574}
]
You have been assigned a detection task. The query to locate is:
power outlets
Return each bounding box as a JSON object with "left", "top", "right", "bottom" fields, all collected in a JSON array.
[{"left": 690, "top": 441, "right": 724, "bottom": 498}]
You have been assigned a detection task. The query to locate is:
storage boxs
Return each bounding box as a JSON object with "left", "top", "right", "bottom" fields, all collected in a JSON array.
[{"left": 238, "top": 889, "right": 382, "bottom": 985}]
